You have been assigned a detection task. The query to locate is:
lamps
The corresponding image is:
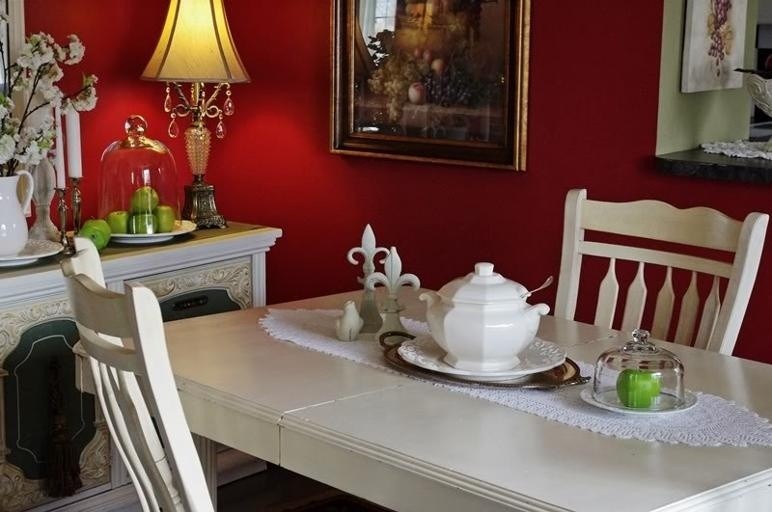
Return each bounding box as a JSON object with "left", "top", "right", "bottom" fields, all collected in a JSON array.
[{"left": 141, "top": 0, "right": 251, "bottom": 229}]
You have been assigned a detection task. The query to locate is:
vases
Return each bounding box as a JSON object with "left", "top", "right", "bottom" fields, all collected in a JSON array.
[{"left": 0, "top": 171, "right": 33, "bottom": 258}]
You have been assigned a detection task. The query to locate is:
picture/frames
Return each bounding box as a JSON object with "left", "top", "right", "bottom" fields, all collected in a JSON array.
[{"left": 328, "top": 0, "right": 531, "bottom": 173}]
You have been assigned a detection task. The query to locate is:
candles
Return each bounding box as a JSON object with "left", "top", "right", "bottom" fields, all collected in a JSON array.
[
  {"left": 66, "top": 111, "right": 83, "bottom": 179},
  {"left": 56, "top": 107, "right": 66, "bottom": 189}
]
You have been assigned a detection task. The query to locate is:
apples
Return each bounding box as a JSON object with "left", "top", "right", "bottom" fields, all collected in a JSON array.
[
  {"left": 431, "top": 59, "right": 447, "bottom": 75},
  {"left": 615, "top": 368, "right": 660, "bottom": 409},
  {"left": 407, "top": 83, "right": 424, "bottom": 104},
  {"left": 77, "top": 186, "right": 172, "bottom": 253}
]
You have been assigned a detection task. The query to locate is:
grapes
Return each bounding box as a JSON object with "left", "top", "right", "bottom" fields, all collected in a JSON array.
[
  {"left": 424, "top": 71, "right": 506, "bottom": 109},
  {"left": 367, "top": 58, "right": 429, "bottom": 124}
]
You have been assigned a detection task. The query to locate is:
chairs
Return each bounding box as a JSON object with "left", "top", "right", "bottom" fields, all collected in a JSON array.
[
  {"left": 60, "top": 239, "right": 217, "bottom": 512},
  {"left": 554, "top": 188, "right": 769, "bottom": 357}
]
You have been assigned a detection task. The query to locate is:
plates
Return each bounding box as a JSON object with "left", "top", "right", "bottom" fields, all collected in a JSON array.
[
  {"left": 109, "top": 219, "right": 197, "bottom": 244},
  {"left": 0, "top": 238, "right": 65, "bottom": 267},
  {"left": 580, "top": 386, "right": 699, "bottom": 417},
  {"left": 396, "top": 334, "right": 569, "bottom": 383}
]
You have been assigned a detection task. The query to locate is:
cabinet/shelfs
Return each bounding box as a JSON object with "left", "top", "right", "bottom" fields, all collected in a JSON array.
[{"left": 0, "top": 222, "right": 282, "bottom": 512}]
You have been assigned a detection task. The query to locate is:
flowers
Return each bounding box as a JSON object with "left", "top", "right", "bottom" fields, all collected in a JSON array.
[{"left": 0, "top": 9, "right": 99, "bottom": 174}]
